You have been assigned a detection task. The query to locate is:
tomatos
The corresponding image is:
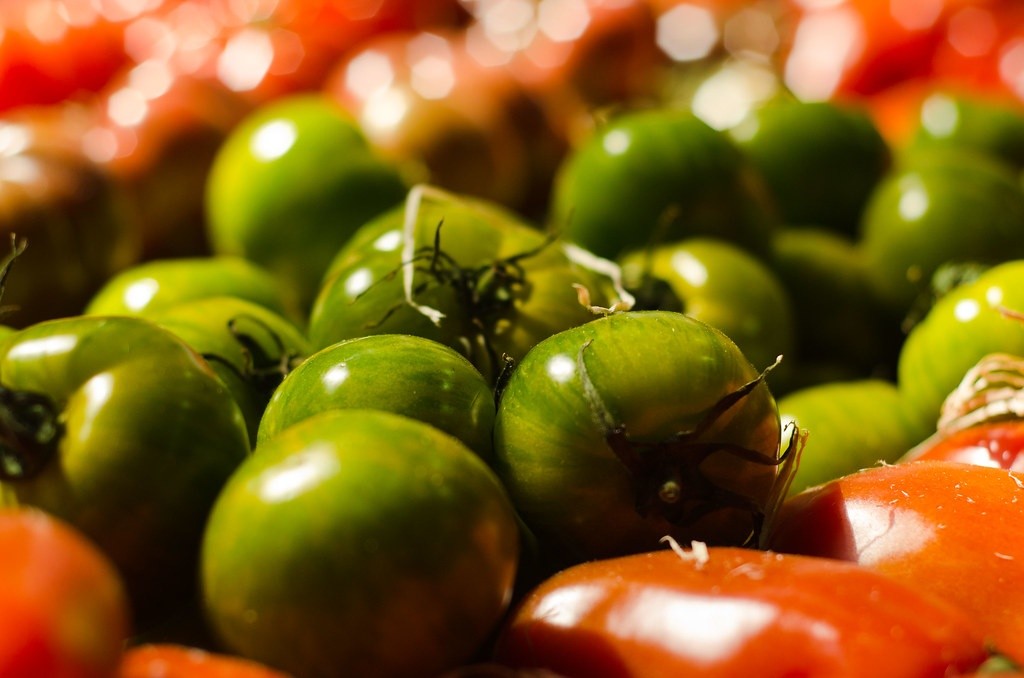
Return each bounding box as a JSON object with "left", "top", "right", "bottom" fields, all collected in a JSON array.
[{"left": 0, "top": 0, "right": 1024, "bottom": 678}]
